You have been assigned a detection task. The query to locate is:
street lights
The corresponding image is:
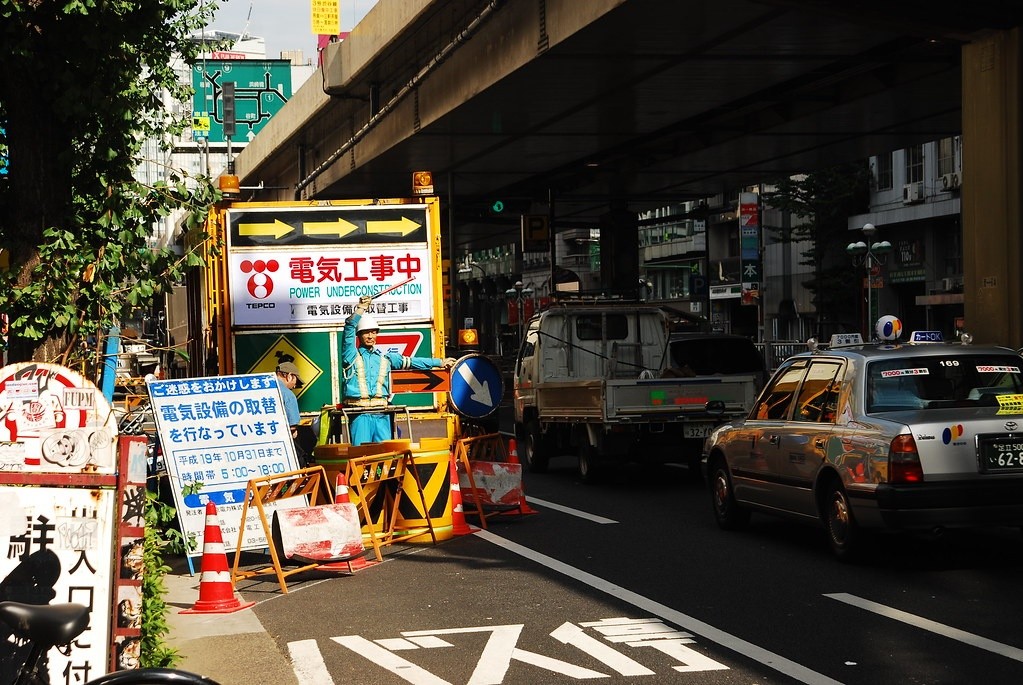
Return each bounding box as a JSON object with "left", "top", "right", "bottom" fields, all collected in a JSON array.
[{"left": 846, "top": 223, "right": 892, "bottom": 343}]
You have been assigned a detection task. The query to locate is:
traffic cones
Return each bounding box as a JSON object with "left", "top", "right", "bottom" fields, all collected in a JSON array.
[
  {"left": 500, "top": 440, "right": 538, "bottom": 515},
  {"left": 179, "top": 500, "right": 256, "bottom": 615},
  {"left": 314, "top": 474, "right": 379, "bottom": 569},
  {"left": 434, "top": 453, "right": 482, "bottom": 535}
]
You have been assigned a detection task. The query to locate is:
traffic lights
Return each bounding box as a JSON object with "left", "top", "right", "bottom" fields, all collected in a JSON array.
[{"left": 488, "top": 199, "right": 510, "bottom": 217}]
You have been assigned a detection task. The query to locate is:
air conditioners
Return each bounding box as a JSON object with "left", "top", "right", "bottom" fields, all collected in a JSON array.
[
  {"left": 943, "top": 173, "right": 954, "bottom": 189},
  {"left": 953, "top": 171, "right": 962, "bottom": 189},
  {"left": 942, "top": 278, "right": 953, "bottom": 291},
  {"left": 903, "top": 183, "right": 918, "bottom": 204}
]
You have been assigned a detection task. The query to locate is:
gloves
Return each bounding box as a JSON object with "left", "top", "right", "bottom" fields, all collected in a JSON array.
[
  {"left": 441, "top": 357, "right": 456, "bottom": 368},
  {"left": 356, "top": 296, "right": 372, "bottom": 311}
]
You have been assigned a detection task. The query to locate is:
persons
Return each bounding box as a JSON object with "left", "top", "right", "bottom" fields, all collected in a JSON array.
[
  {"left": 275, "top": 362, "right": 305, "bottom": 440},
  {"left": 342, "top": 297, "right": 458, "bottom": 445}
]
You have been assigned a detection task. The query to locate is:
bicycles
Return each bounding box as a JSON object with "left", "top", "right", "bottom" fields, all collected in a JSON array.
[{"left": 2, "top": 601, "right": 222, "bottom": 685}]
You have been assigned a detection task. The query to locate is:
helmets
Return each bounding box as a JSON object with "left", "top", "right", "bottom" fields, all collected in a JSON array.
[{"left": 356, "top": 318, "right": 380, "bottom": 335}]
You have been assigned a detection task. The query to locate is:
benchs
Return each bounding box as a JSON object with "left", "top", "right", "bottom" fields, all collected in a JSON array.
[{"left": 867, "top": 385, "right": 1015, "bottom": 413}]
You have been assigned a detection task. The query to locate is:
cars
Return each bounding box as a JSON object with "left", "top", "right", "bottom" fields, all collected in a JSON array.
[{"left": 701, "top": 338, "right": 1023, "bottom": 566}]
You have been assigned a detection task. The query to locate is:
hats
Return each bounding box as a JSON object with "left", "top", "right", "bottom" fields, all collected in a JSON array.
[{"left": 276, "top": 362, "right": 306, "bottom": 385}]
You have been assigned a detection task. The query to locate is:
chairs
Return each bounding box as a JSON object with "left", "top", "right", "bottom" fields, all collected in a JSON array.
[
  {"left": 939, "top": 358, "right": 976, "bottom": 400},
  {"left": 868, "top": 361, "right": 922, "bottom": 404}
]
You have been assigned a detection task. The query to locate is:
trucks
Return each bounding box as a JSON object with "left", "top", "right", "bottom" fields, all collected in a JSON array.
[{"left": 514, "top": 292, "right": 758, "bottom": 483}]
